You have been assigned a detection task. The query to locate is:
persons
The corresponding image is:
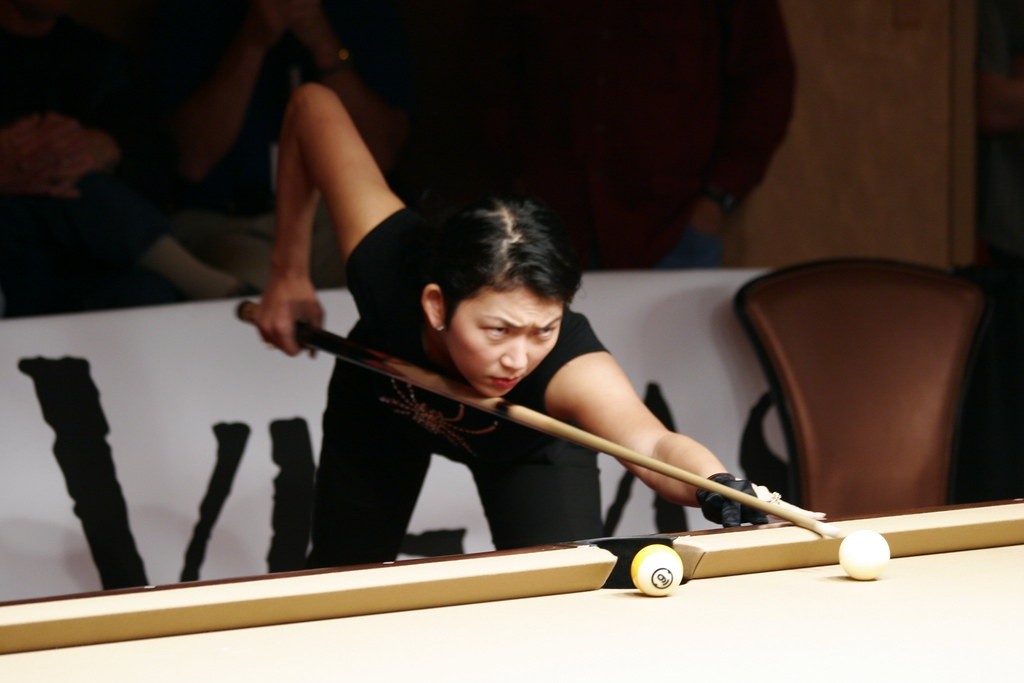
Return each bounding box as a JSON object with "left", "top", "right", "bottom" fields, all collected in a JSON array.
[
  {"left": 256, "top": 80, "right": 828, "bottom": 569},
  {"left": 953, "top": 0, "right": 1024, "bottom": 509},
  {"left": 409, "top": 0, "right": 800, "bottom": 269},
  {"left": 0, "top": 0, "right": 452, "bottom": 312}
]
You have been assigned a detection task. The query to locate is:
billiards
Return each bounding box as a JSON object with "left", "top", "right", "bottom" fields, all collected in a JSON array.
[
  {"left": 631, "top": 544, "right": 684, "bottom": 597},
  {"left": 839, "top": 530, "right": 890, "bottom": 581}
]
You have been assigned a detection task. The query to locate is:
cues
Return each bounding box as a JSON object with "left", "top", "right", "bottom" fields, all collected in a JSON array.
[{"left": 237, "top": 300, "right": 845, "bottom": 540}]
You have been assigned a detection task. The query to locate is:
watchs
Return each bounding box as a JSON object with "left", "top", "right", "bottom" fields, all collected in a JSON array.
[{"left": 704, "top": 191, "right": 737, "bottom": 213}]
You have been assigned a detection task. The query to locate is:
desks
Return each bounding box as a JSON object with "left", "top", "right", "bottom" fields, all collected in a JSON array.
[{"left": 0, "top": 499, "right": 1024, "bottom": 683}]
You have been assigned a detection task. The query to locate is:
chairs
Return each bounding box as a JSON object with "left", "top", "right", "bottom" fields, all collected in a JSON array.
[{"left": 732, "top": 257, "right": 992, "bottom": 522}]
